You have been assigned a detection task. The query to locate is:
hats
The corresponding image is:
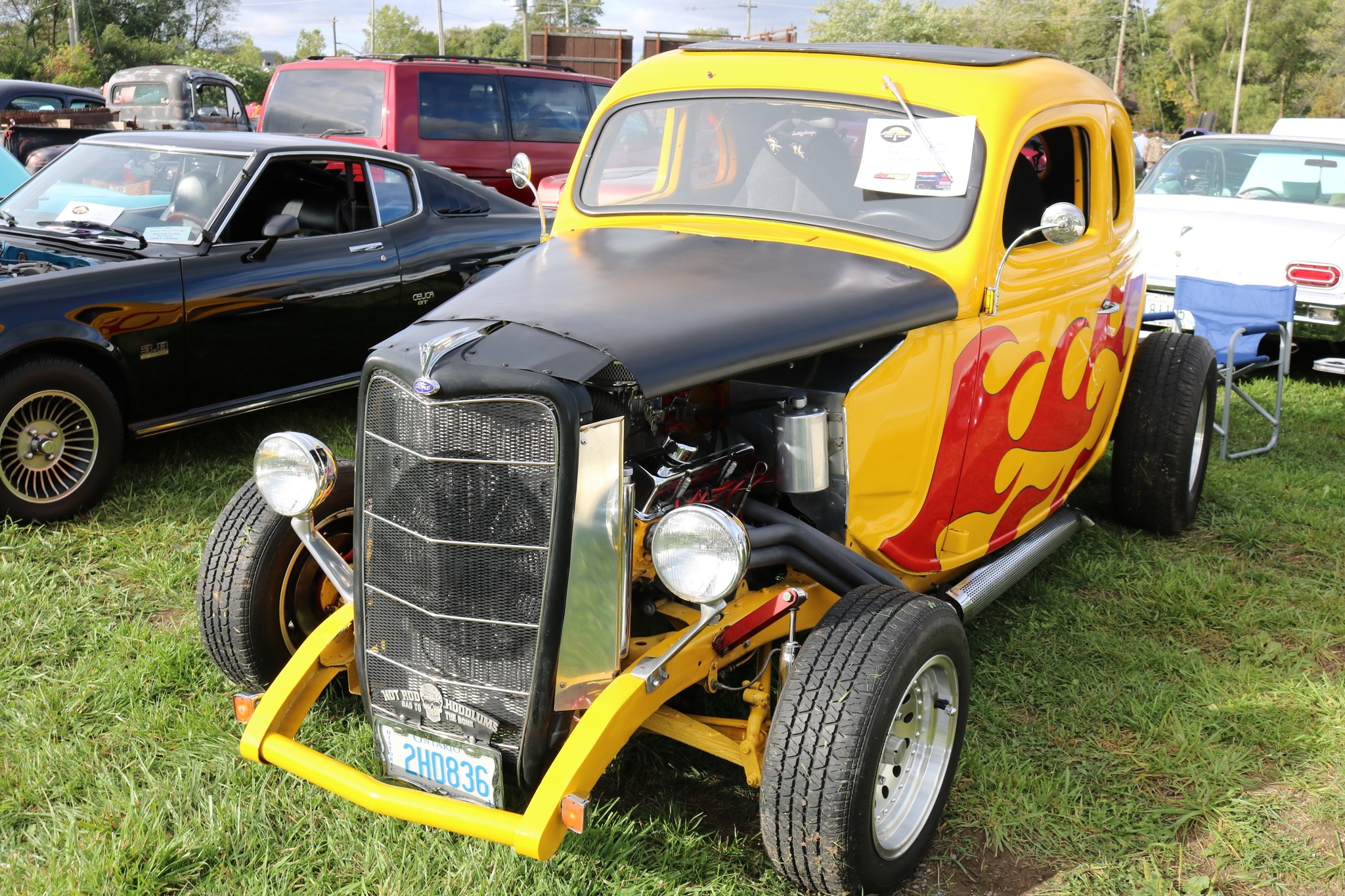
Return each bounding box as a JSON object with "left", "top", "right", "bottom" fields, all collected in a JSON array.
[
  {"left": 1154, "top": 129, "right": 1161, "bottom": 134},
  {"left": 1140, "top": 129, "right": 1148, "bottom": 134}
]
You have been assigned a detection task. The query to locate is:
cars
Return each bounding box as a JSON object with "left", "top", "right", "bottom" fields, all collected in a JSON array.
[
  {"left": 0, "top": 64, "right": 659, "bottom": 528},
  {"left": 1133, "top": 115, "right": 1344, "bottom": 376},
  {"left": 198, "top": 43, "right": 1218, "bottom": 895}
]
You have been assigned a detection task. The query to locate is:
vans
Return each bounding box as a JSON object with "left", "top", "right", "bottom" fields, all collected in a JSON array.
[{"left": 258, "top": 54, "right": 665, "bottom": 210}]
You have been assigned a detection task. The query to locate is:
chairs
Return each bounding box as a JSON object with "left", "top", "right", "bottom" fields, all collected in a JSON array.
[
  {"left": 1003, "top": 152, "right": 1046, "bottom": 249},
  {"left": 159, "top": 170, "right": 223, "bottom": 222},
  {"left": 731, "top": 124, "right": 862, "bottom": 219},
  {"left": 1282, "top": 181, "right": 1322, "bottom": 204},
  {"left": 281, "top": 165, "right": 356, "bottom": 239},
  {"left": 1140, "top": 275, "right": 1296, "bottom": 460}
]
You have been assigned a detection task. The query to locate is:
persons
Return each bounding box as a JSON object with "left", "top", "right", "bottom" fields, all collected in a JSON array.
[
  {"left": 1144, "top": 128, "right": 1168, "bottom": 170},
  {"left": 1133, "top": 128, "right": 1150, "bottom": 165}
]
